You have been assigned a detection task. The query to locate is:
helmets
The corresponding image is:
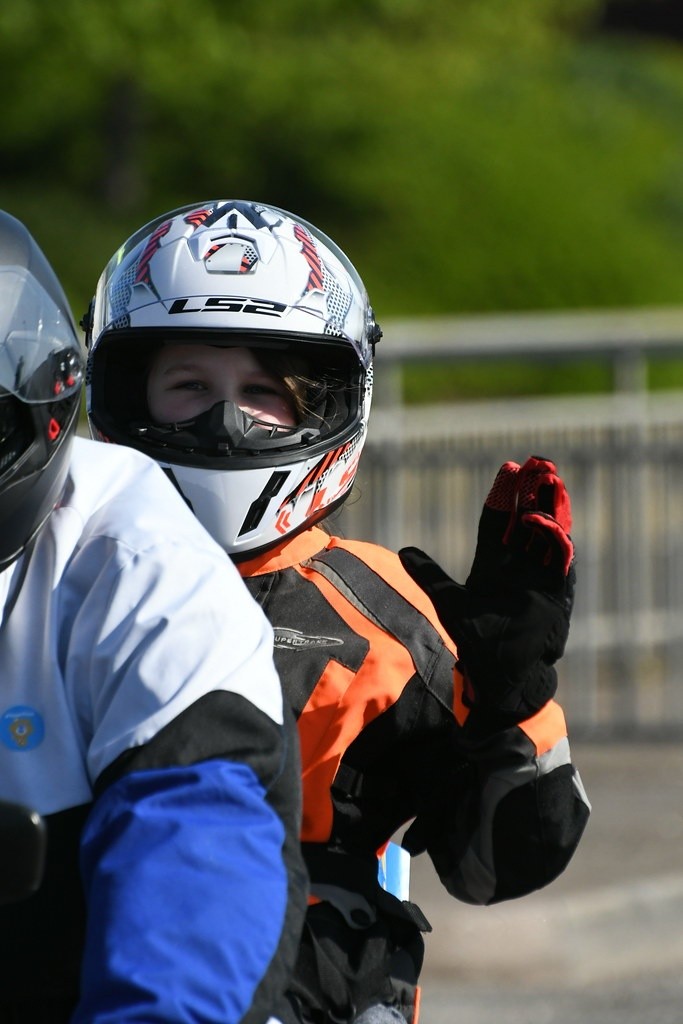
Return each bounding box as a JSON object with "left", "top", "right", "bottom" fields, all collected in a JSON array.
[
  {"left": 0, "top": 210, "right": 84, "bottom": 572},
  {"left": 78, "top": 198, "right": 382, "bottom": 564}
]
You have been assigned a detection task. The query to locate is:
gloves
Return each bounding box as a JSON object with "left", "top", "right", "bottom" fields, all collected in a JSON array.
[{"left": 398, "top": 455, "right": 575, "bottom": 723}]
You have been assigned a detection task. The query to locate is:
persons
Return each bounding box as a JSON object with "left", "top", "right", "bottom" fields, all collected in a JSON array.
[
  {"left": 80, "top": 198, "right": 591, "bottom": 1024},
  {"left": 0, "top": 209, "right": 310, "bottom": 1024}
]
